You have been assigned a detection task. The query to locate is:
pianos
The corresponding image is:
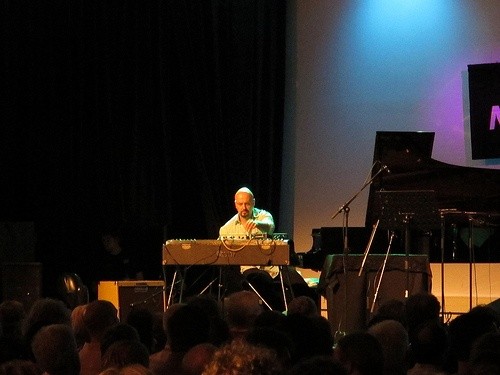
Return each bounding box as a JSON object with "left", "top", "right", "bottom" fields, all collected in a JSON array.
[{"left": 290, "top": 130, "right": 499, "bottom": 269}]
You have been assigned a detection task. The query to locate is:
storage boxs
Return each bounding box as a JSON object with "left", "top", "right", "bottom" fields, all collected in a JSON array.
[
  {"left": 98, "top": 280, "right": 166, "bottom": 326},
  {"left": 317, "top": 254, "right": 432, "bottom": 344}
]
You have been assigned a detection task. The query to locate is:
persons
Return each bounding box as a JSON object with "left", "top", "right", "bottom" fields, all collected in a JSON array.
[
  {"left": 90, "top": 227, "right": 143, "bottom": 294},
  {"left": 0, "top": 268, "right": 500, "bottom": 375},
  {"left": 218, "top": 186, "right": 315, "bottom": 300}
]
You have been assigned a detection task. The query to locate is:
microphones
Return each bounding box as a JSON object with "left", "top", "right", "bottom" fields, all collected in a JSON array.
[{"left": 379, "top": 161, "right": 391, "bottom": 175}]
